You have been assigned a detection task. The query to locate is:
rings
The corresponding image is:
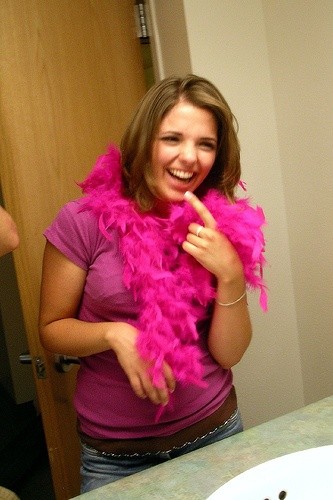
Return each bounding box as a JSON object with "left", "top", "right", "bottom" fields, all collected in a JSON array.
[{"left": 197, "top": 226, "right": 204, "bottom": 237}]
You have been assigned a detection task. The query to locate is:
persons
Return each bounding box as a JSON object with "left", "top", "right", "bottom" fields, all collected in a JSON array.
[
  {"left": 38, "top": 73, "right": 270, "bottom": 494},
  {"left": 0, "top": 206, "right": 20, "bottom": 257}
]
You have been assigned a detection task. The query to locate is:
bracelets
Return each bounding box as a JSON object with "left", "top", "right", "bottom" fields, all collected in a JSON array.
[{"left": 215, "top": 289, "right": 247, "bottom": 307}]
